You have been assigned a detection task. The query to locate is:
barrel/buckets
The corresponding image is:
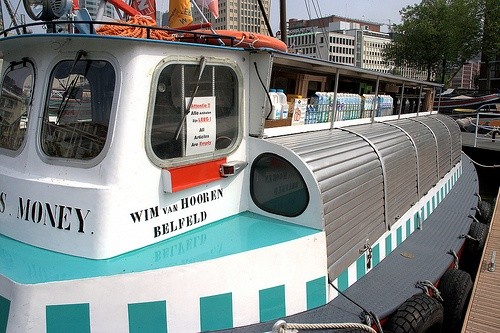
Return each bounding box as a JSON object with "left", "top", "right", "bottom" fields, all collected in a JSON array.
[
  {"left": 276, "top": 89, "right": 289, "bottom": 120},
  {"left": 265, "top": 89, "right": 279, "bottom": 120}
]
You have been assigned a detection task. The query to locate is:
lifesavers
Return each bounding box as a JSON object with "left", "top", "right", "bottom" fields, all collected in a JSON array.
[
  {"left": 476, "top": 201, "right": 491, "bottom": 224},
  {"left": 180, "top": 30, "right": 287, "bottom": 51},
  {"left": 385, "top": 293, "right": 444, "bottom": 333},
  {"left": 460, "top": 222, "right": 489, "bottom": 281},
  {"left": 438, "top": 269, "right": 473, "bottom": 333}
]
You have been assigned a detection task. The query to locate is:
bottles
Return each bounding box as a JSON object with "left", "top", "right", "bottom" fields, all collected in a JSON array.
[{"left": 305, "top": 92, "right": 393, "bottom": 126}]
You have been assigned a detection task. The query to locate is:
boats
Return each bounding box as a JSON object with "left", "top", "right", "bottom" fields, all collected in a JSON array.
[
  {"left": 0, "top": 16, "right": 482, "bottom": 333},
  {"left": 434, "top": 86, "right": 500, "bottom": 114}
]
